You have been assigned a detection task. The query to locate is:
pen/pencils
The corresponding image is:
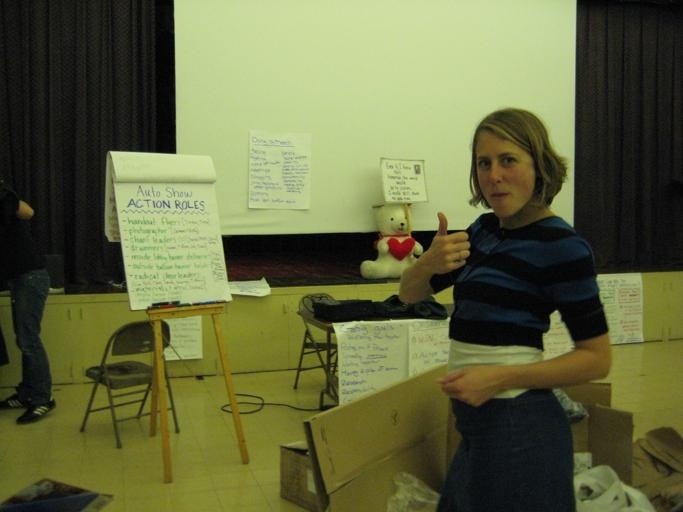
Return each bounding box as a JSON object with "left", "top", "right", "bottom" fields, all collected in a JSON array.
[{"left": 148, "top": 300, "right": 229, "bottom": 309}]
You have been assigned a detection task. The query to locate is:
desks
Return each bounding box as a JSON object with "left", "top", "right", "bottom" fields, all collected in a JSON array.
[{"left": 296, "top": 304, "right": 463, "bottom": 408}]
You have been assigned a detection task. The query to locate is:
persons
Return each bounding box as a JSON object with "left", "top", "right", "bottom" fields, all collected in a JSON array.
[
  {"left": 398, "top": 107, "right": 612, "bottom": 509},
  {"left": 0, "top": 182, "right": 56, "bottom": 426}
]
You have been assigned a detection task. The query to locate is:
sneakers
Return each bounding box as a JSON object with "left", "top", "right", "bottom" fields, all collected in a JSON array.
[
  {"left": 14, "top": 395, "right": 57, "bottom": 425},
  {"left": 0, "top": 391, "right": 26, "bottom": 411}
]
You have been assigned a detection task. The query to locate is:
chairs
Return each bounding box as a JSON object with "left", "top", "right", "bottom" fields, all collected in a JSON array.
[
  {"left": 294, "top": 294, "right": 337, "bottom": 390},
  {"left": 81, "top": 321, "right": 180, "bottom": 448}
]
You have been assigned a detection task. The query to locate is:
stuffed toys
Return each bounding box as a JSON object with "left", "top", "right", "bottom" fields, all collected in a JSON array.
[{"left": 357, "top": 200, "right": 425, "bottom": 281}]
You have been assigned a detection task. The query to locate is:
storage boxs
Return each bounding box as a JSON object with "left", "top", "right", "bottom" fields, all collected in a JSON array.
[{"left": 281, "top": 440, "right": 330, "bottom": 512}]
